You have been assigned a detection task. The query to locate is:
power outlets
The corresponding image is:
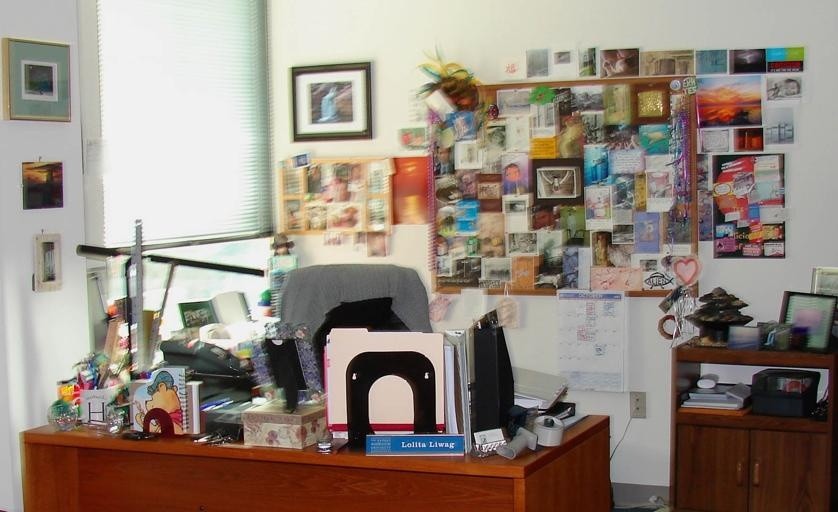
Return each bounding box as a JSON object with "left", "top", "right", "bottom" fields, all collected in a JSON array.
[{"left": 629, "top": 391, "right": 647, "bottom": 418}]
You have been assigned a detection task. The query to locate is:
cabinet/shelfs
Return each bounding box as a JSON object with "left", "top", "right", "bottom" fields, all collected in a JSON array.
[{"left": 667, "top": 348, "right": 838, "bottom": 512}]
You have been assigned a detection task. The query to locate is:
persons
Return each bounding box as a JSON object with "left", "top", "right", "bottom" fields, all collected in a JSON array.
[
  {"left": 767, "top": 77, "right": 802, "bottom": 102},
  {"left": 531, "top": 202, "right": 562, "bottom": 228},
  {"left": 431, "top": 143, "right": 454, "bottom": 176},
  {"left": 260, "top": 233, "right": 300, "bottom": 306}
]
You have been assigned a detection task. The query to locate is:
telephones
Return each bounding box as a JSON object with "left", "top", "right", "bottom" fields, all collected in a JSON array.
[{"left": 161, "top": 339, "right": 240, "bottom": 374}]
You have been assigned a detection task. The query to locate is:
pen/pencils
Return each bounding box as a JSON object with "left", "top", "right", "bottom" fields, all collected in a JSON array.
[
  {"left": 93, "top": 367, "right": 109, "bottom": 390},
  {"left": 199, "top": 397, "right": 234, "bottom": 411},
  {"left": 334, "top": 441, "right": 348, "bottom": 451}
]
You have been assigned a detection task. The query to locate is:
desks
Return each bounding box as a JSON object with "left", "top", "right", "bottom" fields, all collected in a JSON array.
[{"left": 23, "top": 415, "right": 610, "bottom": 511}]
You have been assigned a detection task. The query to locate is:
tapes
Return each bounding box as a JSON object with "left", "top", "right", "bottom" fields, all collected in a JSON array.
[{"left": 532, "top": 416, "right": 564, "bottom": 447}]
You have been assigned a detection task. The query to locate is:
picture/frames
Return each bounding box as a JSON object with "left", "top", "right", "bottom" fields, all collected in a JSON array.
[
  {"left": 288, "top": 62, "right": 372, "bottom": 141},
  {"left": 33, "top": 232, "right": 63, "bottom": 292},
  {"left": 0, "top": 37, "right": 71, "bottom": 122},
  {"left": 428, "top": 75, "right": 699, "bottom": 295}
]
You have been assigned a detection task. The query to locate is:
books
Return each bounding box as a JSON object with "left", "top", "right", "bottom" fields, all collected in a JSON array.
[
  {"left": 321, "top": 324, "right": 476, "bottom": 459},
  {"left": 680, "top": 383, "right": 751, "bottom": 411},
  {"left": 510, "top": 365, "right": 570, "bottom": 411}
]
